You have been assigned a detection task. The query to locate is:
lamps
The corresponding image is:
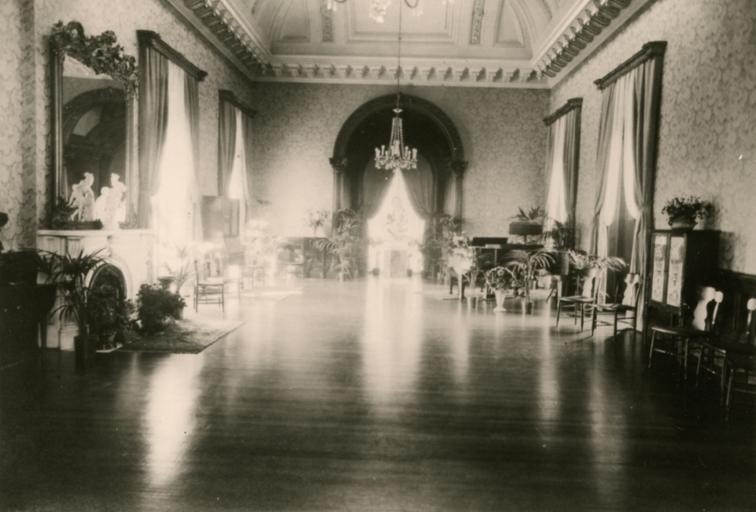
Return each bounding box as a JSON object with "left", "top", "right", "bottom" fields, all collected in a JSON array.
[{"left": 373, "top": 1, "right": 424, "bottom": 171}]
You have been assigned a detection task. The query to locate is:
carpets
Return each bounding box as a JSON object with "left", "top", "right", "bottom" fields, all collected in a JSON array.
[{"left": 119, "top": 319, "right": 242, "bottom": 354}]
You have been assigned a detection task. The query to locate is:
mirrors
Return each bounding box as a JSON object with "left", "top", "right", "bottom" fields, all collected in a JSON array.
[{"left": 49, "top": 19, "right": 139, "bottom": 228}]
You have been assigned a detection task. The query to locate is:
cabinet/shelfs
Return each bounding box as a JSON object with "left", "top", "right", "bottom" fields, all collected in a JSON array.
[
  {"left": 645, "top": 228, "right": 724, "bottom": 346},
  {"left": 293, "top": 236, "right": 327, "bottom": 279}
]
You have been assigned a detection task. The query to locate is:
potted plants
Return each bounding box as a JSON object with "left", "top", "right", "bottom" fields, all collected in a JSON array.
[
  {"left": 511, "top": 251, "right": 556, "bottom": 314},
  {"left": 22, "top": 243, "right": 108, "bottom": 374},
  {"left": 662, "top": 196, "right": 707, "bottom": 229},
  {"left": 485, "top": 267, "right": 512, "bottom": 309}
]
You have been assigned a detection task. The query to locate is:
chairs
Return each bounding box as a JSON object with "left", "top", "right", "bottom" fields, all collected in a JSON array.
[
  {"left": 646, "top": 282, "right": 732, "bottom": 379},
  {"left": 194, "top": 261, "right": 227, "bottom": 311},
  {"left": 590, "top": 278, "right": 643, "bottom": 341},
  {"left": 555, "top": 272, "right": 598, "bottom": 336},
  {"left": 693, "top": 284, "right": 756, "bottom": 422},
  {"left": 449, "top": 247, "right": 484, "bottom": 311}
]
